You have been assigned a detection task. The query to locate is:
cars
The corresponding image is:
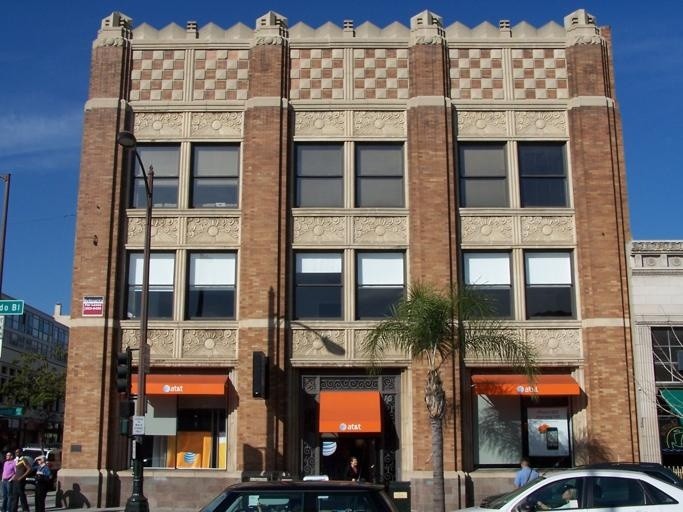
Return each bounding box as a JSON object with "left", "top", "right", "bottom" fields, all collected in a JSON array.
[
  {"left": 199, "top": 481, "right": 400, "bottom": 512},
  {"left": 453, "top": 462, "right": 683, "bottom": 512},
  {"left": 21, "top": 447, "right": 62, "bottom": 484}
]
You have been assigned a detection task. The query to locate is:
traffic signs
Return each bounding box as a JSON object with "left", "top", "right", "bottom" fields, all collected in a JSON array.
[{"left": 0, "top": 299, "right": 25, "bottom": 317}]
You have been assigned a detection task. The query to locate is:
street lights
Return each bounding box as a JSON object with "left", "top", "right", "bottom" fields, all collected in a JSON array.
[{"left": 116, "top": 130, "right": 155, "bottom": 511}]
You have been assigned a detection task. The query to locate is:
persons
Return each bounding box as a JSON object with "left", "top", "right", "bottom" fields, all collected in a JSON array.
[
  {"left": 535, "top": 484, "right": 578, "bottom": 510},
  {"left": 33, "top": 454, "right": 52, "bottom": 512},
  {"left": 0, "top": 451, "right": 16, "bottom": 512},
  {"left": 344, "top": 455, "right": 361, "bottom": 483},
  {"left": 512, "top": 458, "right": 539, "bottom": 489},
  {"left": 10, "top": 447, "right": 32, "bottom": 512}
]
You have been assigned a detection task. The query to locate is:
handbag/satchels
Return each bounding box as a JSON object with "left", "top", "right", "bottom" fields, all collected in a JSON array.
[{"left": 35, "top": 475, "right": 54, "bottom": 489}]
